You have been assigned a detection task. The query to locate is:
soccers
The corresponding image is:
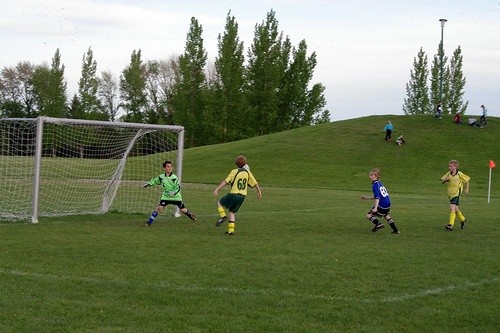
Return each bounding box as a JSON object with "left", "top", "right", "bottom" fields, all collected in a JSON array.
[{"left": 243, "top": 164, "right": 250, "bottom": 171}]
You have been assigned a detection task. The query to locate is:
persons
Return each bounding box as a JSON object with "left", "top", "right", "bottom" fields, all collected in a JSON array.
[
  {"left": 440, "top": 159, "right": 471, "bottom": 231},
  {"left": 467, "top": 117, "right": 484, "bottom": 128},
  {"left": 361, "top": 168, "right": 400, "bottom": 234},
  {"left": 213, "top": 156, "right": 262, "bottom": 236},
  {"left": 480, "top": 105, "right": 487, "bottom": 126},
  {"left": 434, "top": 101, "right": 444, "bottom": 120},
  {"left": 140, "top": 161, "right": 200, "bottom": 227},
  {"left": 452, "top": 113, "right": 462, "bottom": 124},
  {"left": 383, "top": 119, "right": 394, "bottom": 141}
]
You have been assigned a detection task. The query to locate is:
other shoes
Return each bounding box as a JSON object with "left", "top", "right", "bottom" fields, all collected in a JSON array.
[
  {"left": 144, "top": 221, "right": 150, "bottom": 227},
  {"left": 191, "top": 215, "right": 197, "bottom": 220},
  {"left": 215, "top": 216, "right": 227, "bottom": 227},
  {"left": 391, "top": 230, "right": 400, "bottom": 234},
  {"left": 372, "top": 224, "right": 384, "bottom": 232},
  {"left": 445, "top": 224, "right": 454, "bottom": 231},
  {"left": 460, "top": 219, "right": 466, "bottom": 229},
  {"left": 225, "top": 231, "right": 233, "bottom": 236}
]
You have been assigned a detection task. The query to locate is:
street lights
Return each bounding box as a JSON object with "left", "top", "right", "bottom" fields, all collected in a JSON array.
[{"left": 438, "top": 18, "right": 448, "bottom": 106}]
